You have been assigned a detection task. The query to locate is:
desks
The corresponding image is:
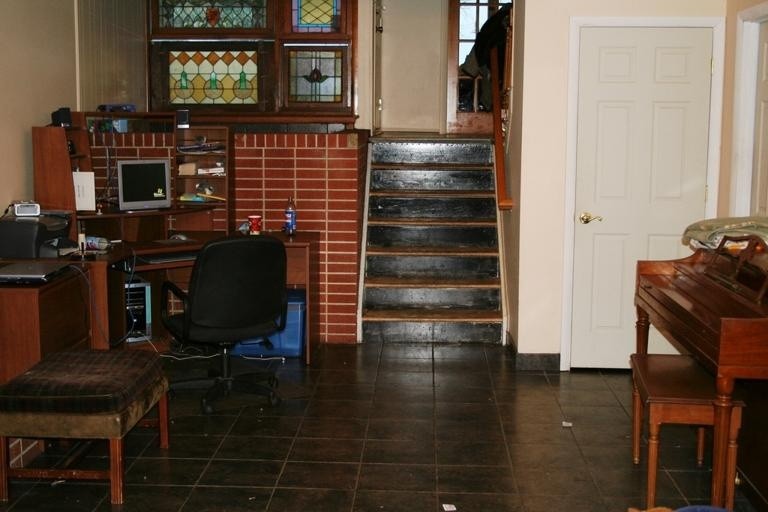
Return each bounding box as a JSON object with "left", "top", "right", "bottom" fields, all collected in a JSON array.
[{"left": 30, "top": 116, "right": 320, "bottom": 369}]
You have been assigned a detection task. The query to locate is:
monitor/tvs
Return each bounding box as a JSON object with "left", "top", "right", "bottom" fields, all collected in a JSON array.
[{"left": 117, "top": 160, "right": 171, "bottom": 214}]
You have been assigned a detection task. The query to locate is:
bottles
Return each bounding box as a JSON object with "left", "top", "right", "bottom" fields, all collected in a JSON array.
[
  {"left": 85, "top": 237, "right": 111, "bottom": 249},
  {"left": 285, "top": 196, "right": 296, "bottom": 236}
]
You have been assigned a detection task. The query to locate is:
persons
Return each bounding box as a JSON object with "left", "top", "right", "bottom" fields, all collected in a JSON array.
[{"left": 474, "top": 3, "right": 512, "bottom": 112}]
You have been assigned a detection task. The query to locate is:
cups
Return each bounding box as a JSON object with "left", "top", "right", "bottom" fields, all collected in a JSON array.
[{"left": 248, "top": 215, "right": 262, "bottom": 235}]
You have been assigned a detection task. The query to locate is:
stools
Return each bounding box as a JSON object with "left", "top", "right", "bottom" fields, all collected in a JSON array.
[
  {"left": 0, "top": 340, "right": 174, "bottom": 510},
  {"left": 622, "top": 350, "right": 744, "bottom": 511}
]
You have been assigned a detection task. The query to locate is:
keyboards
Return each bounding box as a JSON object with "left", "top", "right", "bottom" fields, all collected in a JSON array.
[{"left": 135, "top": 251, "right": 198, "bottom": 264}]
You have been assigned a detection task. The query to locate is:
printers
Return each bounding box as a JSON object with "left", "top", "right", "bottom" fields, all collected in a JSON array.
[{"left": 0, "top": 209, "right": 73, "bottom": 258}]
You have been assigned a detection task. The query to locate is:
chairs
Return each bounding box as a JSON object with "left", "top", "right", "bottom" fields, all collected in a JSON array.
[{"left": 151, "top": 227, "right": 290, "bottom": 419}]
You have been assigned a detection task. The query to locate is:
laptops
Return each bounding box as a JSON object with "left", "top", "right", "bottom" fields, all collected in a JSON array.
[{"left": 0, "top": 264, "right": 72, "bottom": 285}]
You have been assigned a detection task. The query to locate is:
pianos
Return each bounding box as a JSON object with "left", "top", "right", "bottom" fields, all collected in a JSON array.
[{"left": 631, "top": 235, "right": 768, "bottom": 512}]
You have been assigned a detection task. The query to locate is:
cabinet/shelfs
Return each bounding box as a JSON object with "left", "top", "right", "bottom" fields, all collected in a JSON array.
[{"left": 0, "top": 253, "right": 95, "bottom": 463}]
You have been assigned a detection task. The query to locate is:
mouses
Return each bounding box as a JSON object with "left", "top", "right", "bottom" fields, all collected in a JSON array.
[{"left": 169, "top": 234, "right": 187, "bottom": 241}]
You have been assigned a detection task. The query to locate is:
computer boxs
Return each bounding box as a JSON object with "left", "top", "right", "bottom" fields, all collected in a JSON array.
[{"left": 124, "top": 272, "right": 152, "bottom": 342}]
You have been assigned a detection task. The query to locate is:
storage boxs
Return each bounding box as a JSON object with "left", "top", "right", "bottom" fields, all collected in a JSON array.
[{"left": 228, "top": 287, "right": 305, "bottom": 360}]
